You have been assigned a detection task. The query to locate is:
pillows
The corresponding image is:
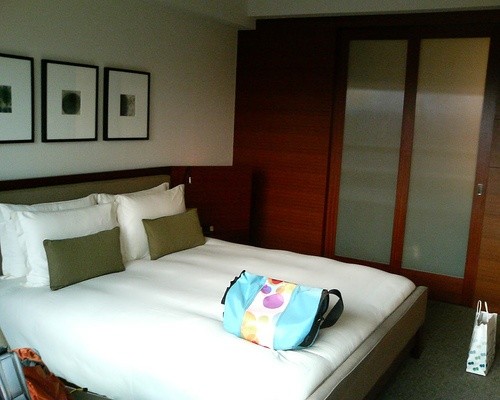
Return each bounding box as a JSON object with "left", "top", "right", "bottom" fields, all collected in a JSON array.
[
  {"left": 19, "top": 203, "right": 122, "bottom": 289},
  {"left": 99, "top": 181, "right": 170, "bottom": 215},
  {"left": 116, "top": 184, "right": 186, "bottom": 263},
  {"left": 43, "top": 225, "right": 126, "bottom": 290},
  {"left": 0, "top": 195, "right": 102, "bottom": 277},
  {"left": 142, "top": 207, "right": 207, "bottom": 262}
]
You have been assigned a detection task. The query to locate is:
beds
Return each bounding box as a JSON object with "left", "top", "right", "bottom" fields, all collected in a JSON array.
[{"left": 0, "top": 174, "right": 431, "bottom": 398}]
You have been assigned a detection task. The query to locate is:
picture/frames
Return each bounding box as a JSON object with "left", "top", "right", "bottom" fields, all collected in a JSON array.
[
  {"left": 0, "top": 54, "right": 34, "bottom": 144},
  {"left": 102, "top": 67, "right": 151, "bottom": 140},
  {"left": 40, "top": 58, "right": 100, "bottom": 143}
]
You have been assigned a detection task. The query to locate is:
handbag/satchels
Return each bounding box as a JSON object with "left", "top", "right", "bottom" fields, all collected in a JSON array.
[
  {"left": 221, "top": 268, "right": 345, "bottom": 353},
  {"left": 465, "top": 299, "right": 498, "bottom": 378}
]
